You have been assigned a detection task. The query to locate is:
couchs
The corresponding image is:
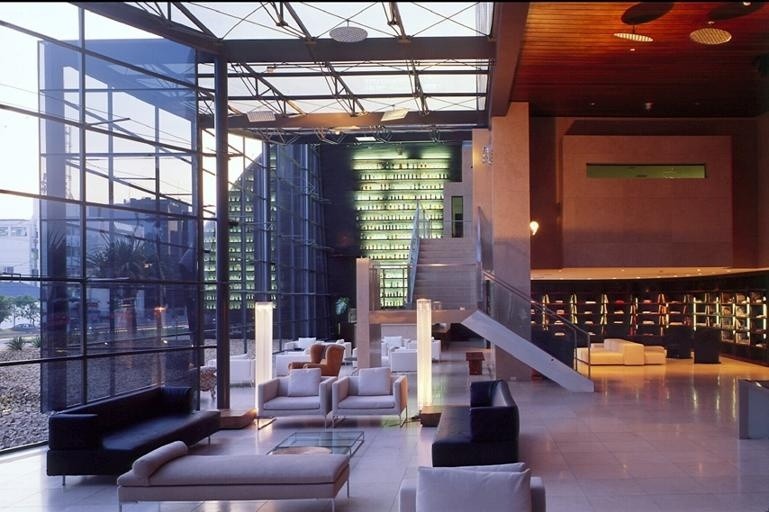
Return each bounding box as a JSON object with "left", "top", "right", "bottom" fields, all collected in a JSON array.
[
  {"left": 47, "top": 386, "right": 221, "bottom": 486},
  {"left": 431, "top": 380, "right": 519, "bottom": 467},
  {"left": 531, "top": 320, "right": 723, "bottom": 370}
]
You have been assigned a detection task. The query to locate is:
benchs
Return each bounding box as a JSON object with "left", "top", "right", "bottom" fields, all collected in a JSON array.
[{"left": 117, "top": 440, "right": 350, "bottom": 512}]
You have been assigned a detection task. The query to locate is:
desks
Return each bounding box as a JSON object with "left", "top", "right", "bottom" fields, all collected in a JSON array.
[
  {"left": 266, "top": 432, "right": 363, "bottom": 461},
  {"left": 466, "top": 351, "right": 486, "bottom": 376}
]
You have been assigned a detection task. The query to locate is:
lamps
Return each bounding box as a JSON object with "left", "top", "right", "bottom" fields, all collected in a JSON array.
[
  {"left": 612, "top": 25, "right": 655, "bottom": 46},
  {"left": 381, "top": 103, "right": 409, "bottom": 121},
  {"left": 328, "top": 18, "right": 367, "bottom": 43},
  {"left": 246, "top": 110, "right": 275, "bottom": 123},
  {"left": 529, "top": 220, "right": 539, "bottom": 238},
  {"left": 689, "top": 18, "right": 733, "bottom": 48}
]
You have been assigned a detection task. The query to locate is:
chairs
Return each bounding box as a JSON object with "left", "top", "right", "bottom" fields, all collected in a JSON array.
[
  {"left": 165, "top": 339, "right": 217, "bottom": 400},
  {"left": 275, "top": 334, "right": 441, "bottom": 377},
  {"left": 207, "top": 354, "right": 255, "bottom": 387},
  {"left": 255, "top": 376, "right": 335, "bottom": 430},
  {"left": 398, "top": 477, "right": 546, "bottom": 512},
  {"left": 740, "top": 379, "right": 768, "bottom": 440},
  {"left": 332, "top": 374, "right": 408, "bottom": 428}
]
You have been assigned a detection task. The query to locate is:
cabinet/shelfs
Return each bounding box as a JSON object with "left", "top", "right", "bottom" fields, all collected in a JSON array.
[{"left": 532, "top": 289, "right": 769, "bottom": 368}]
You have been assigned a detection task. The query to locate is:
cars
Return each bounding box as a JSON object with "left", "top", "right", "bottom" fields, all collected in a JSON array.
[
  {"left": 66, "top": 316, "right": 100, "bottom": 345},
  {"left": 7, "top": 323, "right": 40, "bottom": 331}
]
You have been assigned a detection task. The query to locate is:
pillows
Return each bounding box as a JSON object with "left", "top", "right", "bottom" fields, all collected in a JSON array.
[
  {"left": 359, "top": 367, "right": 391, "bottom": 395},
  {"left": 462, "top": 462, "right": 527, "bottom": 472},
  {"left": 415, "top": 466, "right": 532, "bottom": 512},
  {"left": 287, "top": 368, "right": 322, "bottom": 397}
]
